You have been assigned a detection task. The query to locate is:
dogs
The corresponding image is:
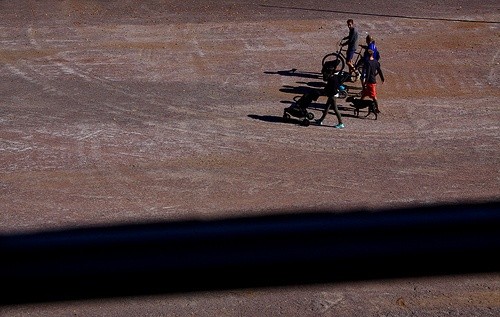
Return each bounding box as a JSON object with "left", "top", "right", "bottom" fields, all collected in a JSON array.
[{"left": 345, "top": 94, "right": 381, "bottom": 121}]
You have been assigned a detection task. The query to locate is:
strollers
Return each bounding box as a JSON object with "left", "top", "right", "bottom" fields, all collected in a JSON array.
[
  {"left": 282, "top": 88, "right": 325, "bottom": 127},
  {"left": 326, "top": 65, "right": 356, "bottom": 98}
]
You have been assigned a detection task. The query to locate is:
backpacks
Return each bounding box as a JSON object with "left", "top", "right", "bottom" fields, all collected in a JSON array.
[{"left": 376, "top": 49, "right": 381, "bottom": 61}]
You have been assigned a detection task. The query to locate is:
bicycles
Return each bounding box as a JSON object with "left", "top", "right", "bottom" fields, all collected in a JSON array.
[{"left": 321, "top": 39, "right": 379, "bottom": 76}]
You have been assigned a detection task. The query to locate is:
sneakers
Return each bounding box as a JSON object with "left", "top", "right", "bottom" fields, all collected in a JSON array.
[
  {"left": 312, "top": 120, "right": 322, "bottom": 126},
  {"left": 335, "top": 123, "right": 345, "bottom": 129}
]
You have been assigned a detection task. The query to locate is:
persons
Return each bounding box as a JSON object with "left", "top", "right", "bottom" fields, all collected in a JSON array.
[
  {"left": 360, "top": 50, "right": 384, "bottom": 113},
  {"left": 314, "top": 65, "right": 344, "bottom": 128},
  {"left": 340, "top": 19, "right": 361, "bottom": 82},
  {"left": 358, "top": 34, "right": 376, "bottom": 77}
]
